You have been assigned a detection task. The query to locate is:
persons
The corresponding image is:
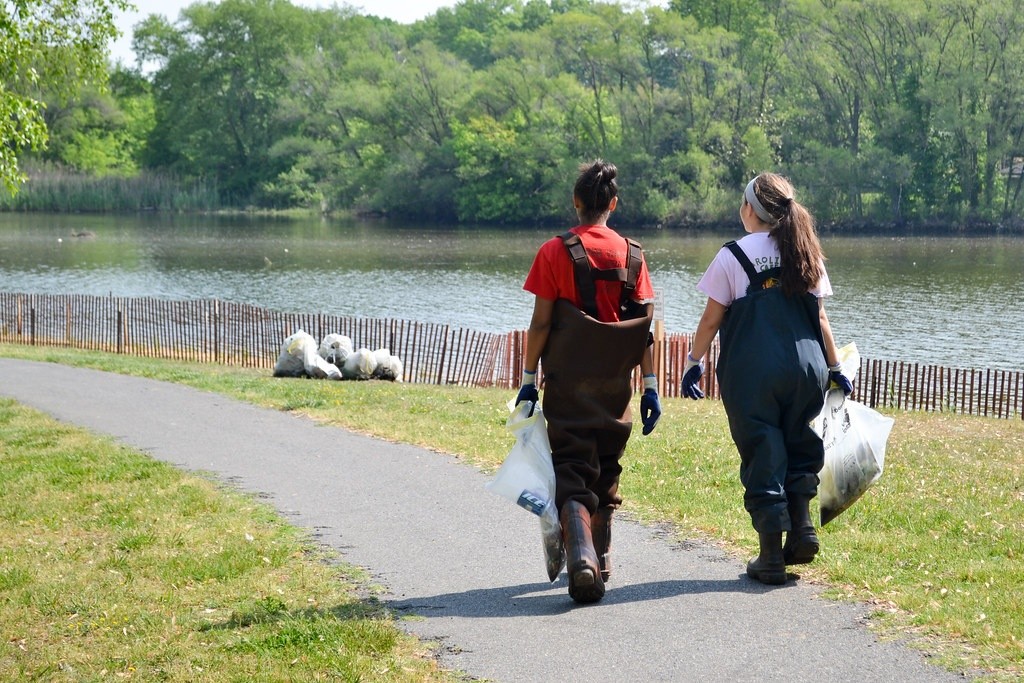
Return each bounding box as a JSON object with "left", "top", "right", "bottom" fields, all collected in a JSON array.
[
  {"left": 516, "top": 161, "right": 662, "bottom": 605},
  {"left": 681, "top": 172, "right": 852, "bottom": 584}
]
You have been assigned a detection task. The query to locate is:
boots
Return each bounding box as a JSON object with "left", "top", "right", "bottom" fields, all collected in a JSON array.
[
  {"left": 560, "top": 499, "right": 605, "bottom": 603},
  {"left": 590, "top": 509, "right": 611, "bottom": 582},
  {"left": 782, "top": 496, "right": 819, "bottom": 566},
  {"left": 746, "top": 532, "right": 788, "bottom": 584}
]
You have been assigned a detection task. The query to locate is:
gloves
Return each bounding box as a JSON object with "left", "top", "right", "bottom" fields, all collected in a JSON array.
[
  {"left": 827, "top": 363, "right": 855, "bottom": 396},
  {"left": 681, "top": 353, "right": 705, "bottom": 400},
  {"left": 514, "top": 372, "right": 538, "bottom": 417},
  {"left": 640, "top": 374, "right": 660, "bottom": 435}
]
